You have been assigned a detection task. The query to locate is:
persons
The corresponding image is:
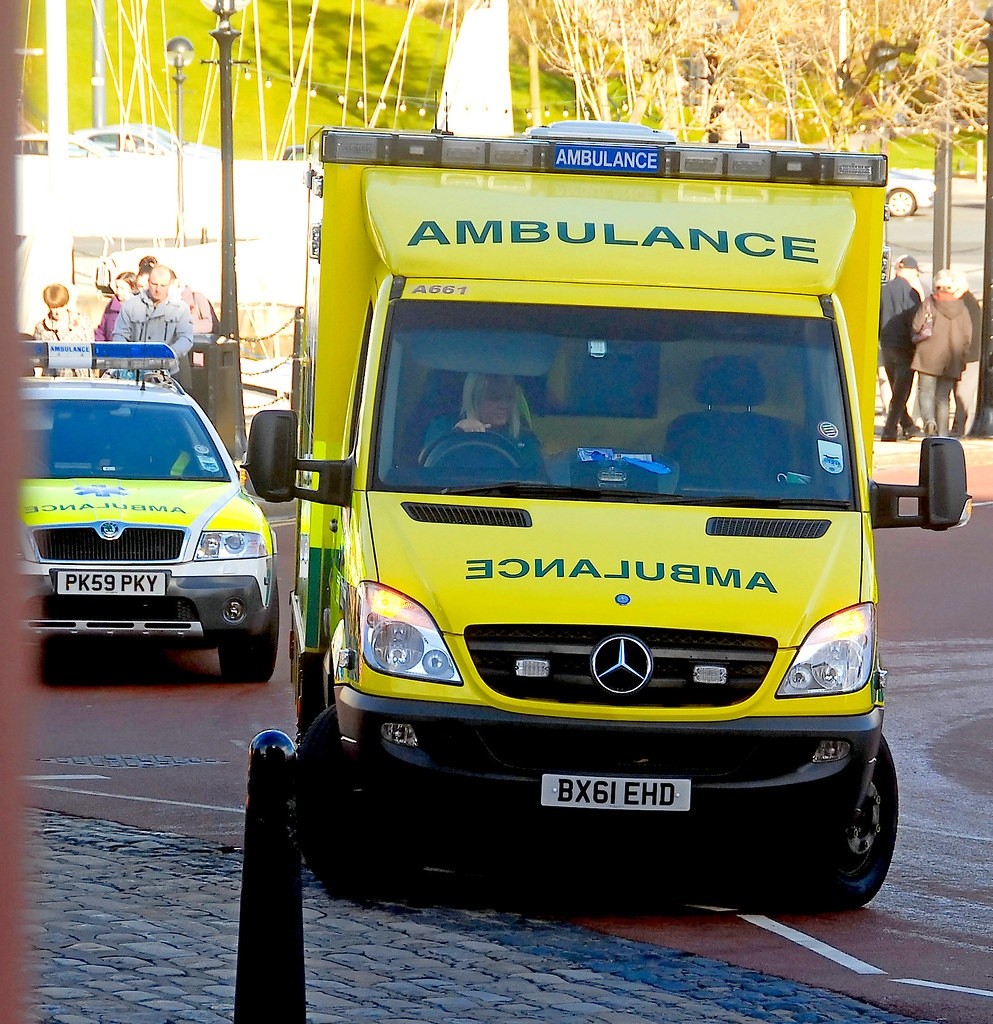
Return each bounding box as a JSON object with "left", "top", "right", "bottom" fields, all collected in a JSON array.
[
  {"left": 880, "top": 254, "right": 980, "bottom": 440},
  {"left": 421, "top": 352, "right": 550, "bottom": 484},
  {"left": 33, "top": 256, "right": 219, "bottom": 392}
]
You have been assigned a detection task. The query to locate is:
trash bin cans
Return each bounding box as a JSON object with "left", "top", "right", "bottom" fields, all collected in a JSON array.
[{"left": 187, "top": 332, "right": 241, "bottom": 464}]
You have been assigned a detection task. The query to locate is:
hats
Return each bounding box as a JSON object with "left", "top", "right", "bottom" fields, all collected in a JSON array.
[{"left": 899, "top": 257, "right": 922, "bottom": 273}]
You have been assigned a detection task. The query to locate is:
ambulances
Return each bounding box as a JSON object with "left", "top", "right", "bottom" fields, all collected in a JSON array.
[
  {"left": 248, "top": 120, "right": 974, "bottom": 913},
  {"left": 15, "top": 338, "right": 280, "bottom": 684}
]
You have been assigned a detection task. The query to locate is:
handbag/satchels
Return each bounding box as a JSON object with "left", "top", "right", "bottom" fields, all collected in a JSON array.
[{"left": 912, "top": 300, "right": 932, "bottom": 343}]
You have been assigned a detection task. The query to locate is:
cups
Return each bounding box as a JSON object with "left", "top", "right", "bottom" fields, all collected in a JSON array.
[{"left": 777, "top": 472, "right": 812, "bottom": 484}]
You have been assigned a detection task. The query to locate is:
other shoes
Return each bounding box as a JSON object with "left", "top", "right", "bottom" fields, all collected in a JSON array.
[
  {"left": 881, "top": 433, "right": 898, "bottom": 440},
  {"left": 927, "top": 424, "right": 935, "bottom": 435},
  {"left": 903, "top": 424, "right": 920, "bottom": 435}
]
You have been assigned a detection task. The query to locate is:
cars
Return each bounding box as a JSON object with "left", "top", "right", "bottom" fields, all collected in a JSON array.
[
  {"left": 13, "top": 123, "right": 323, "bottom": 249},
  {"left": 886, "top": 167, "right": 938, "bottom": 218}
]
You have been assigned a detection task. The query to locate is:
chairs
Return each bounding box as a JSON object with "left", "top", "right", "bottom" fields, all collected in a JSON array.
[{"left": 665, "top": 354, "right": 791, "bottom": 487}]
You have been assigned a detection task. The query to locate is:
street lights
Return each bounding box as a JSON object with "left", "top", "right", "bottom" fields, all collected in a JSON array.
[
  {"left": 200, "top": 0, "right": 250, "bottom": 469},
  {"left": 164, "top": 36, "right": 196, "bottom": 249}
]
